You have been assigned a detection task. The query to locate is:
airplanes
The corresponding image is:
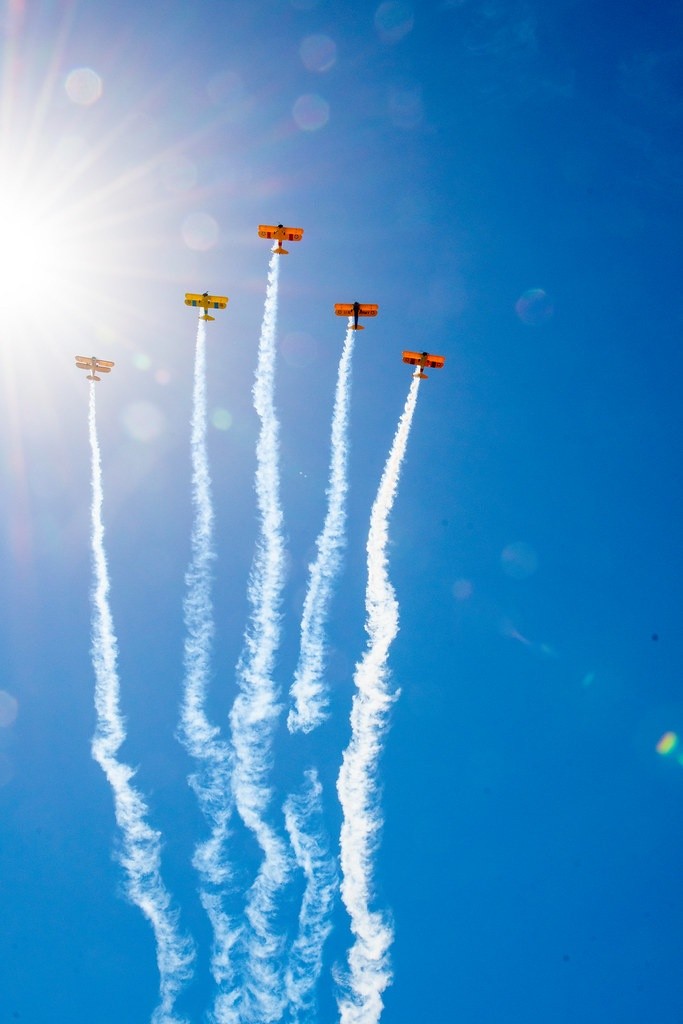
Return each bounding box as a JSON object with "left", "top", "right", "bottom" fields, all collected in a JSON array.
[{"left": 72, "top": 220, "right": 443, "bottom": 383}]
